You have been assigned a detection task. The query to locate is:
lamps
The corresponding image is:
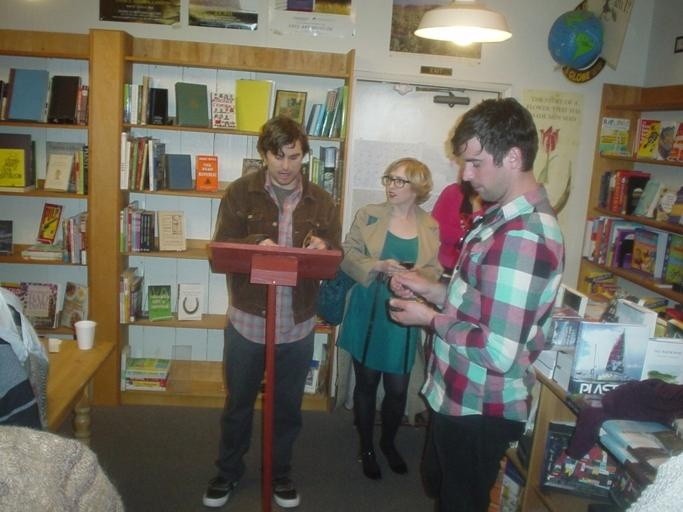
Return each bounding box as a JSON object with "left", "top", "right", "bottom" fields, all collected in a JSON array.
[
  {"left": 413, "top": 1, "right": 512, "bottom": 48},
  {"left": 433, "top": 90, "right": 469, "bottom": 108}
]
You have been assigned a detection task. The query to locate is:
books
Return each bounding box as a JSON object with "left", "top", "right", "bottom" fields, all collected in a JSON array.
[
  {"left": 48, "top": 75, "right": 77, "bottom": 125},
  {"left": 669, "top": 122, "right": 683, "bottom": 161},
  {"left": 273, "top": 90, "right": 307, "bottom": 127},
  {"left": 212, "top": 93, "right": 237, "bottom": 128},
  {"left": 0, "top": 80, "right": 8, "bottom": 121},
  {"left": 236, "top": 80, "right": 274, "bottom": 131},
  {"left": 124, "top": 77, "right": 168, "bottom": 126},
  {"left": 242, "top": 159, "right": 263, "bottom": 178},
  {"left": 590, "top": 413, "right": 681, "bottom": 512},
  {"left": 0, "top": 282, "right": 21, "bottom": 297},
  {"left": 660, "top": 233, "right": 682, "bottom": 291},
  {"left": 21, "top": 202, "right": 87, "bottom": 264},
  {"left": 576, "top": 261, "right": 619, "bottom": 301},
  {"left": 121, "top": 133, "right": 165, "bottom": 193},
  {"left": 60, "top": 282, "right": 88, "bottom": 331},
  {"left": 599, "top": 170, "right": 683, "bottom": 224},
  {"left": 148, "top": 287, "right": 172, "bottom": 321},
  {"left": 640, "top": 339, "right": 683, "bottom": 384},
  {"left": 177, "top": 285, "right": 202, "bottom": 321},
  {"left": 1, "top": 133, "right": 37, "bottom": 192},
  {"left": 490, "top": 456, "right": 525, "bottom": 511},
  {"left": 616, "top": 300, "right": 658, "bottom": 337},
  {"left": 502, "top": 382, "right": 541, "bottom": 468},
  {"left": 300, "top": 145, "right": 343, "bottom": 201},
  {"left": 306, "top": 86, "right": 349, "bottom": 138},
  {"left": 6, "top": 67, "right": 47, "bottom": 123},
  {"left": 632, "top": 226, "right": 668, "bottom": 278},
  {"left": 303, "top": 360, "right": 315, "bottom": 396},
  {"left": 533, "top": 306, "right": 578, "bottom": 391},
  {"left": 121, "top": 267, "right": 144, "bottom": 322},
  {"left": 120, "top": 202, "right": 155, "bottom": 254},
  {"left": 633, "top": 119, "right": 662, "bottom": 160},
  {"left": 44, "top": 140, "right": 89, "bottom": 195},
  {"left": 568, "top": 321, "right": 650, "bottom": 398},
  {"left": 196, "top": 157, "right": 219, "bottom": 193},
  {"left": 541, "top": 420, "right": 618, "bottom": 498},
  {"left": 78, "top": 83, "right": 89, "bottom": 126},
  {"left": 561, "top": 285, "right": 587, "bottom": 315},
  {"left": 158, "top": 210, "right": 187, "bottom": 251},
  {"left": 600, "top": 119, "right": 630, "bottom": 156},
  {"left": 176, "top": 83, "right": 209, "bottom": 128},
  {"left": 165, "top": 154, "right": 194, "bottom": 190},
  {"left": 652, "top": 306, "right": 683, "bottom": 338},
  {"left": 21, "top": 282, "right": 58, "bottom": 329},
  {"left": 583, "top": 216, "right": 643, "bottom": 270},
  {"left": 319, "top": 344, "right": 329, "bottom": 395},
  {"left": 121, "top": 345, "right": 172, "bottom": 391},
  {"left": 0, "top": 221, "right": 13, "bottom": 256},
  {"left": 658, "top": 122, "right": 676, "bottom": 160}
]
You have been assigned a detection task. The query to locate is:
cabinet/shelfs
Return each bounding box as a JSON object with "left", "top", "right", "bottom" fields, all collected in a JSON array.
[
  {"left": 0, "top": 30, "right": 356, "bottom": 412},
  {"left": 576, "top": 80, "right": 683, "bottom": 338},
  {"left": 492, "top": 364, "right": 682, "bottom": 512}
]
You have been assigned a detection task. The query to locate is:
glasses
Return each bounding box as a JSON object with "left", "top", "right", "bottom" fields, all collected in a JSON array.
[{"left": 382, "top": 175, "right": 410, "bottom": 189}]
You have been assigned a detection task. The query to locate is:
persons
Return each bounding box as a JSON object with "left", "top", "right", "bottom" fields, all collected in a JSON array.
[
  {"left": 431, "top": 170, "right": 497, "bottom": 282},
  {"left": 201, "top": 116, "right": 345, "bottom": 507},
  {"left": 341, "top": 157, "right": 444, "bottom": 479},
  {"left": 387, "top": 99, "right": 566, "bottom": 512}
]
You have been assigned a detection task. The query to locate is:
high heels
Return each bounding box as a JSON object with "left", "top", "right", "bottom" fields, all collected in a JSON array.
[
  {"left": 359, "top": 444, "right": 382, "bottom": 481},
  {"left": 383, "top": 439, "right": 408, "bottom": 475}
]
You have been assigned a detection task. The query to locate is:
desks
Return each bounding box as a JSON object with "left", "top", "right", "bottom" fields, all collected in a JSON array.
[{"left": 39, "top": 335, "right": 116, "bottom": 446}]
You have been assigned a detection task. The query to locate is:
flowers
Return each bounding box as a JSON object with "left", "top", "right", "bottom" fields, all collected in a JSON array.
[
  {"left": 551, "top": 132, "right": 579, "bottom": 213},
  {"left": 539, "top": 125, "right": 560, "bottom": 183}
]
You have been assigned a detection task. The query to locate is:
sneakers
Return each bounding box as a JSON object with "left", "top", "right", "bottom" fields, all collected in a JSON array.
[
  {"left": 201, "top": 477, "right": 239, "bottom": 508},
  {"left": 271, "top": 478, "right": 302, "bottom": 510}
]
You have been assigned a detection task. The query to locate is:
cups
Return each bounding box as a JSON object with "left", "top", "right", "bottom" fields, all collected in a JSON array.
[{"left": 74, "top": 320, "right": 98, "bottom": 352}]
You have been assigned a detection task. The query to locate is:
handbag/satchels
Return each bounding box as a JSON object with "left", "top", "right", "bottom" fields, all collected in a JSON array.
[{"left": 316, "top": 216, "right": 378, "bottom": 325}]
